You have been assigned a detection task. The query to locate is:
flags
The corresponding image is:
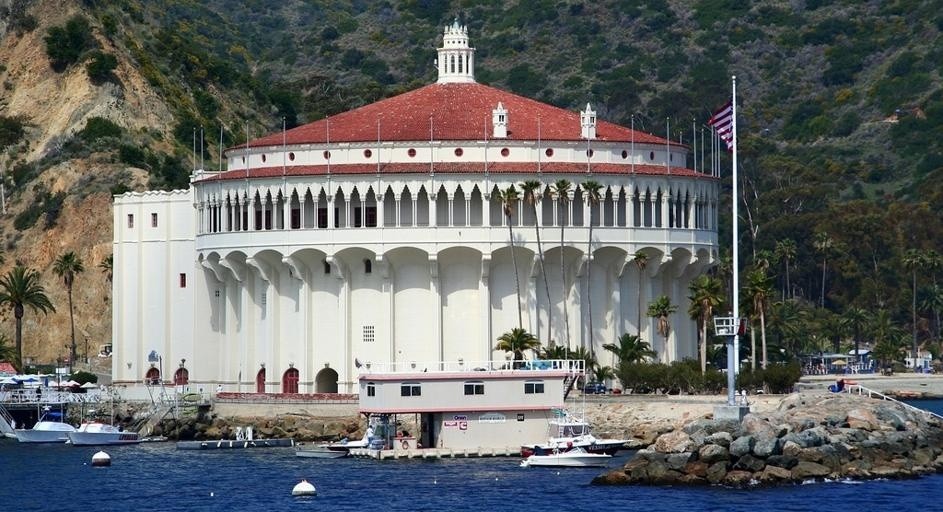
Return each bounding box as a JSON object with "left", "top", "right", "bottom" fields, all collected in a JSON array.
[{"left": 706, "top": 102, "right": 733, "bottom": 152}]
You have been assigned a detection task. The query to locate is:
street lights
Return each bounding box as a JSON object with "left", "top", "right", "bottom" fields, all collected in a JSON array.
[
  {"left": 55, "top": 357, "right": 61, "bottom": 402},
  {"left": 68, "top": 344, "right": 72, "bottom": 375},
  {"left": 83, "top": 337, "right": 88, "bottom": 364}
]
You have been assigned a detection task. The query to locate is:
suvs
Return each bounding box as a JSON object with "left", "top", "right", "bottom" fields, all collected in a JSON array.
[{"left": 580, "top": 381, "right": 606, "bottom": 394}]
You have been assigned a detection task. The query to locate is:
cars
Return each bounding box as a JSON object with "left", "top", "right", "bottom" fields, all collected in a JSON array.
[{"left": 97, "top": 349, "right": 111, "bottom": 358}]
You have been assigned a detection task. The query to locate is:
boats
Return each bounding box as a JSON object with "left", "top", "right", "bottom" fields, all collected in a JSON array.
[
  {"left": 525, "top": 443, "right": 612, "bottom": 470},
  {"left": 520, "top": 383, "right": 634, "bottom": 460},
  {"left": 64, "top": 403, "right": 141, "bottom": 446},
  {"left": 11, "top": 399, "right": 76, "bottom": 443},
  {"left": 294, "top": 442, "right": 347, "bottom": 459},
  {"left": 326, "top": 414, "right": 410, "bottom": 452}
]
